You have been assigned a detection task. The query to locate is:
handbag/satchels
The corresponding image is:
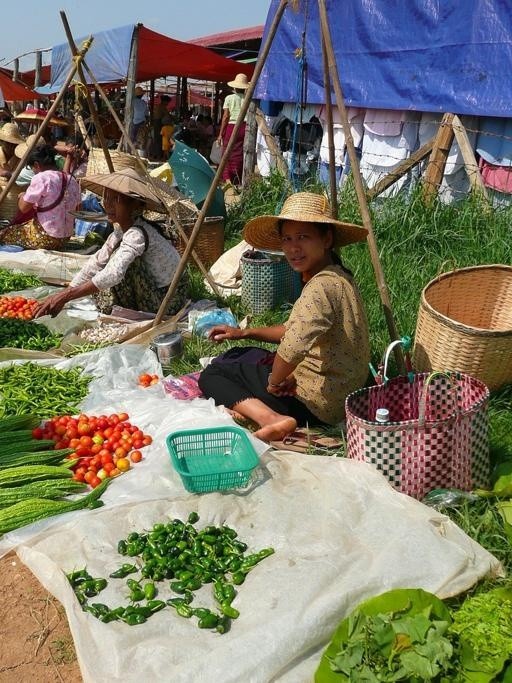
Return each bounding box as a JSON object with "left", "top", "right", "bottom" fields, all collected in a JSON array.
[
  {"left": 209, "top": 137, "right": 224, "bottom": 165},
  {"left": 10, "top": 202, "right": 36, "bottom": 223}
]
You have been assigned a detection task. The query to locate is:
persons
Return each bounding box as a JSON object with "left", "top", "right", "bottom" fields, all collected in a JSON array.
[
  {"left": 216, "top": 74, "right": 250, "bottom": 184},
  {"left": 202, "top": 192, "right": 369, "bottom": 443},
  {"left": 30, "top": 167, "right": 185, "bottom": 319},
  {"left": 0, "top": 86, "right": 221, "bottom": 249}
]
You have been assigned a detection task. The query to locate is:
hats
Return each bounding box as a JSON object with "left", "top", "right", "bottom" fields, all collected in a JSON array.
[
  {"left": 134, "top": 86, "right": 144, "bottom": 95},
  {"left": 226, "top": 73, "right": 251, "bottom": 88},
  {"left": 74, "top": 167, "right": 168, "bottom": 213},
  {"left": 0, "top": 122, "right": 46, "bottom": 158},
  {"left": 241, "top": 192, "right": 369, "bottom": 251}
]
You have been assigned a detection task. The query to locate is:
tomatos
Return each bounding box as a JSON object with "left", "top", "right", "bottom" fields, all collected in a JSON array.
[
  {"left": 0, "top": 296, "right": 39, "bottom": 321},
  {"left": 140, "top": 374, "right": 159, "bottom": 388},
  {"left": 32, "top": 413, "right": 152, "bottom": 488}
]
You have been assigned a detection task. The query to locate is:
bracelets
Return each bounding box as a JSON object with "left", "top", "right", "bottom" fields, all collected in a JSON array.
[{"left": 267, "top": 372, "right": 287, "bottom": 387}]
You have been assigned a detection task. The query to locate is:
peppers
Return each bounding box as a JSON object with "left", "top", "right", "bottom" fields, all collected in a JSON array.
[
  {"left": 66, "top": 512, "right": 275, "bottom": 635},
  {"left": 0, "top": 360, "right": 96, "bottom": 419},
  {"left": 0, "top": 317, "right": 63, "bottom": 352},
  {"left": 0, "top": 266, "right": 46, "bottom": 295}
]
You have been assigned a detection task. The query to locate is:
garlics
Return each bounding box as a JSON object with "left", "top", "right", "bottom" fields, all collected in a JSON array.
[{"left": 82, "top": 322, "right": 128, "bottom": 343}]
